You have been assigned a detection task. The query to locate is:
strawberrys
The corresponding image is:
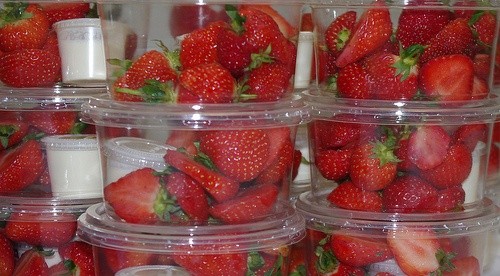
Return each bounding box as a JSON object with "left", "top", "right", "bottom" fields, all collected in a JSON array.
[{"left": 0, "top": 0, "right": 500, "bottom": 276}]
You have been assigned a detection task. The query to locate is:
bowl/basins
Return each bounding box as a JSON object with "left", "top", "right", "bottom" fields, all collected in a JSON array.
[{"left": 0, "top": 0, "right": 500, "bottom": 276}]
